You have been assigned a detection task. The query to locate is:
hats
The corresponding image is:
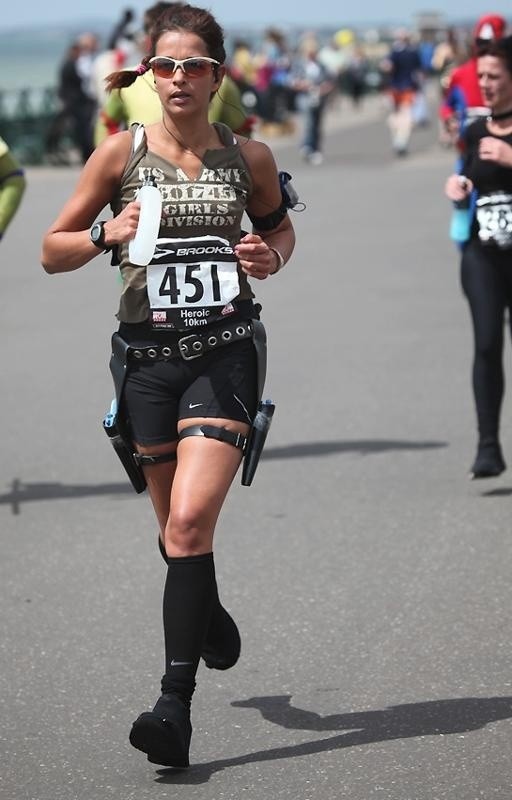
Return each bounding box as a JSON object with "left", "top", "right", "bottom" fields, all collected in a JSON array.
[{"left": 475, "top": 15, "right": 508, "bottom": 39}]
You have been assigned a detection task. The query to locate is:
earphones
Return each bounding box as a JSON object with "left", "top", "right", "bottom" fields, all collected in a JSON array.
[
  {"left": 214, "top": 70, "right": 220, "bottom": 84},
  {"left": 153, "top": 80, "right": 156, "bottom": 85}
]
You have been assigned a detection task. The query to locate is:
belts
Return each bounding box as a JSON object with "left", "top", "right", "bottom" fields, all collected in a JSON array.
[{"left": 108, "top": 320, "right": 257, "bottom": 367}]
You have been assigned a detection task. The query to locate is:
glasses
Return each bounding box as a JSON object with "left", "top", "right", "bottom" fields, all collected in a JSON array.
[{"left": 146, "top": 54, "right": 222, "bottom": 79}]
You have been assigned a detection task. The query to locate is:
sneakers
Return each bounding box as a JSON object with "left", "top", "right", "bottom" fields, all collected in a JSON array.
[
  {"left": 463, "top": 434, "right": 507, "bottom": 483},
  {"left": 126, "top": 691, "right": 196, "bottom": 771},
  {"left": 190, "top": 594, "right": 244, "bottom": 675}
]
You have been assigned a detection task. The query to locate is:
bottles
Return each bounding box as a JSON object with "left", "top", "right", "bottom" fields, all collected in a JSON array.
[{"left": 449, "top": 178, "right": 473, "bottom": 241}]
